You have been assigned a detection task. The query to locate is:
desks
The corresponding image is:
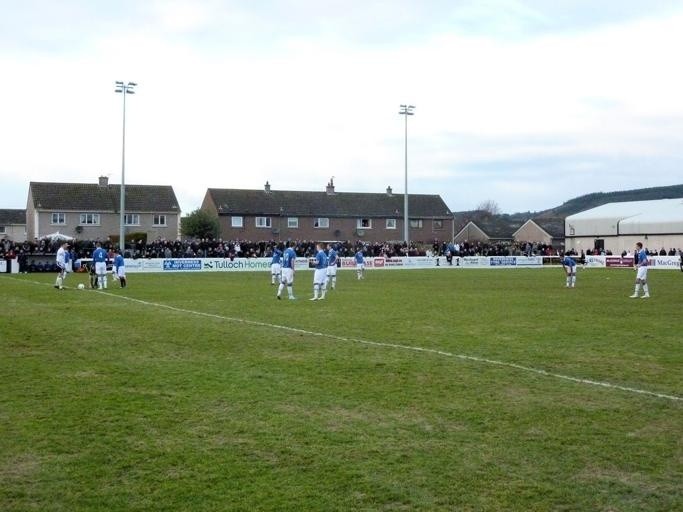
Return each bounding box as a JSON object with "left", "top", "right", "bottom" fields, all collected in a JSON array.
[
  {"left": 399, "top": 105, "right": 415, "bottom": 246},
  {"left": 115, "top": 81, "right": 136, "bottom": 259}
]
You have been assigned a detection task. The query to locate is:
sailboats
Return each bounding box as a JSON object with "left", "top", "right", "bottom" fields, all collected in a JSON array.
[{"left": 78, "top": 283, "right": 84, "bottom": 289}]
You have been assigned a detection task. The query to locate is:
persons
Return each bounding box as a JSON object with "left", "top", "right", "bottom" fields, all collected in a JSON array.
[
  {"left": 52, "top": 240, "right": 126, "bottom": 291},
  {"left": 275, "top": 239, "right": 297, "bottom": 300},
  {"left": 308, "top": 242, "right": 328, "bottom": 300},
  {"left": 352, "top": 247, "right": 367, "bottom": 280},
  {"left": 324, "top": 243, "right": 339, "bottom": 289},
  {"left": 628, "top": 241, "right": 651, "bottom": 299},
  {"left": 268, "top": 242, "right": 283, "bottom": 285},
  {"left": 559, "top": 250, "right": 577, "bottom": 288}
]
